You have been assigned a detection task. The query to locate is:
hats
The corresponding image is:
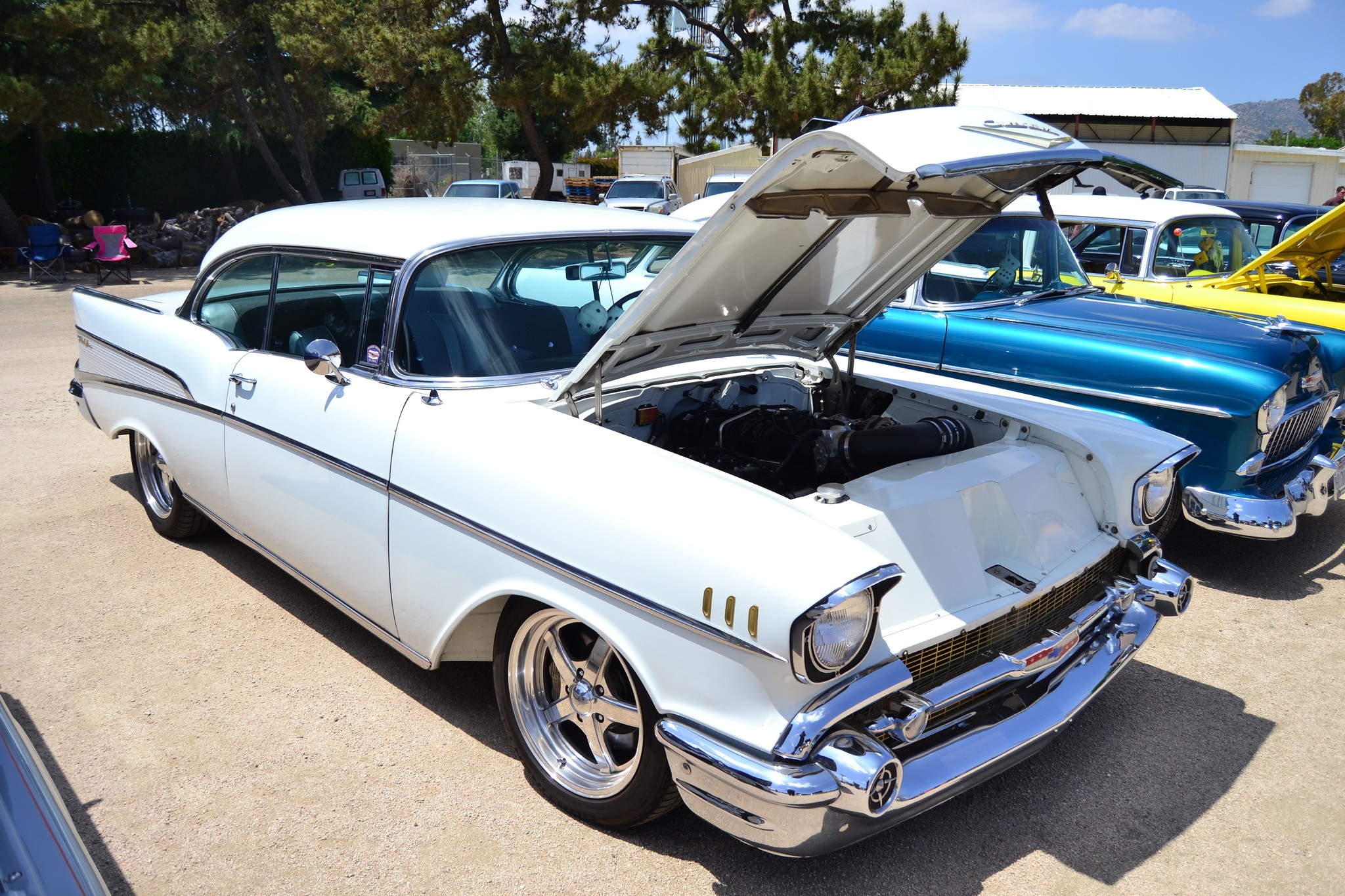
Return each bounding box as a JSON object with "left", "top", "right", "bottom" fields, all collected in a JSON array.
[{"left": 1092, "top": 186, "right": 1106, "bottom": 195}]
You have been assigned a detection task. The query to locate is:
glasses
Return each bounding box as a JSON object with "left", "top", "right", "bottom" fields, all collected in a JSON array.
[{"left": 1340, "top": 192, "right": 1345, "bottom": 194}]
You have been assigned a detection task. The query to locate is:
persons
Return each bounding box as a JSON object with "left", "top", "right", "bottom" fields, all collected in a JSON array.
[
  {"left": 1322, "top": 186, "right": 1345, "bottom": 207},
  {"left": 1071, "top": 186, "right": 1118, "bottom": 245}
]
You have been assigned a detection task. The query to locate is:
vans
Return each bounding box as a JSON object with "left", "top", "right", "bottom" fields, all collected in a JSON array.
[{"left": 338, "top": 168, "right": 387, "bottom": 201}]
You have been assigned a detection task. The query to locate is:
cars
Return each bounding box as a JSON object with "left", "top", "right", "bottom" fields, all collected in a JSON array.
[
  {"left": 497, "top": 170, "right": 1345, "bottom": 543},
  {"left": 63, "top": 103, "right": 1199, "bottom": 863},
  {"left": 443, "top": 180, "right": 523, "bottom": 198}
]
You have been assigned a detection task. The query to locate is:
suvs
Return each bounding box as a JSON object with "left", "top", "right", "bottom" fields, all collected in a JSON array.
[{"left": 597, "top": 176, "right": 684, "bottom": 217}]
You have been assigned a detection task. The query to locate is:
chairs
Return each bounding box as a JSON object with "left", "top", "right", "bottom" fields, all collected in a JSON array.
[
  {"left": 16, "top": 223, "right": 75, "bottom": 286},
  {"left": 82, "top": 225, "right": 136, "bottom": 285}
]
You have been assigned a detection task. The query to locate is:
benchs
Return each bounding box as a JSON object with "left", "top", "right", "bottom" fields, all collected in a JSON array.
[{"left": 232, "top": 291, "right": 506, "bottom": 356}]
